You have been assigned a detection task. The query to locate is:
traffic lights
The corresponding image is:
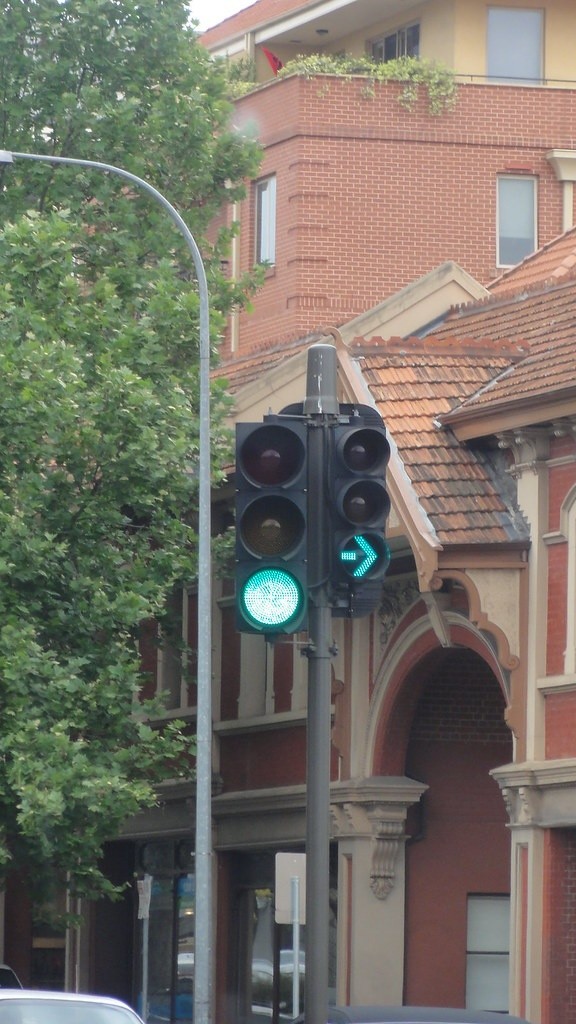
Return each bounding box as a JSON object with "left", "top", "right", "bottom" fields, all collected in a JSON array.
[
  {"left": 235, "top": 419, "right": 313, "bottom": 635},
  {"left": 332, "top": 423, "right": 391, "bottom": 586}
]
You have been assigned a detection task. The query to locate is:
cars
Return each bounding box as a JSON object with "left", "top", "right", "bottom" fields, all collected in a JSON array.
[
  {"left": 286, "top": 1006, "right": 531, "bottom": 1024},
  {"left": 0, "top": 990, "right": 146, "bottom": 1024},
  {"left": 169, "top": 908, "right": 307, "bottom": 1024}
]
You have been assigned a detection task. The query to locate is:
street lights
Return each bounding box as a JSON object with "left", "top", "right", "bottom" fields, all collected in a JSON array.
[{"left": 0, "top": 147, "right": 219, "bottom": 1024}]
